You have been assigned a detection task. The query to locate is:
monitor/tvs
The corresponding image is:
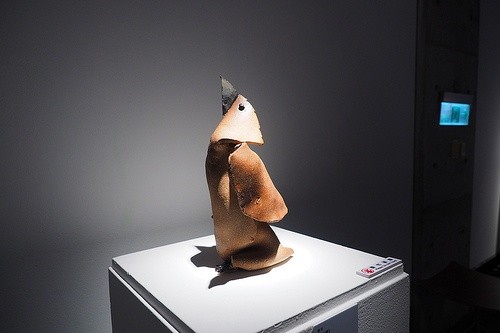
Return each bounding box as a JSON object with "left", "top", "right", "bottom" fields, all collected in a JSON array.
[{"left": 436, "top": 93, "right": 473, "bottom": 143}]
